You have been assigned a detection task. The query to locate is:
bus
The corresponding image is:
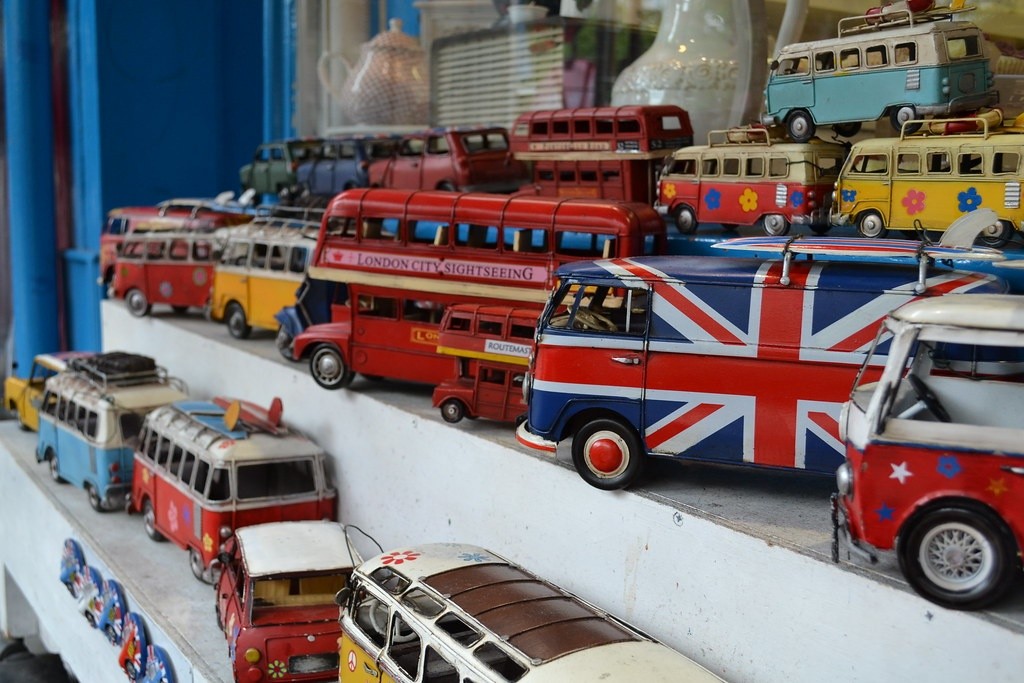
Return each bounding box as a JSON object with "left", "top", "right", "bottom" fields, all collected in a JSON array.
[
  {"left": 208, "top": 518, "right": 370, "bottom": 683},
  {"left": 504, "top": 104, "right": 696, "bottom": 200},
  {"left": 127, "top": 395, "right": 337, "bottom": 587},
  {"left": 514, "top": 231, "right": 1024, "bottom": 494},
  {"left": 826, "top": 107, "right": 1024, "bottom": 250},
  {"left": 828, "top": 289, "right": 1024, "bottom": 615},
  {"left": 653, "top": 127, "right": 853, "bottom": 237},
  {"left": 2, "top": 351, "right": 96, "bottom": 432},
  {"left": 331, "top": 539, "right": 728, "bottom": 683},
  {"left": 94, "top": 119, "right": 669, "bottom": 422},
  {"left": 758, "top": 0, "right": 1001, "bottom": 144},
  {"left": 35, "top": 351, "right": 195, "bottom": 513}
]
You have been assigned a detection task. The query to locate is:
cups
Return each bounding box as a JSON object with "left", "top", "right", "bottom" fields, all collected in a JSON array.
[{"left": 505, "top": 5, "right": 549, "bottom": 25}]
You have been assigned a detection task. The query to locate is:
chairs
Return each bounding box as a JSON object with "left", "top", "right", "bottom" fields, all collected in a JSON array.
[{"left": 363, "top": 218, "right": 616, "bottom": 259}]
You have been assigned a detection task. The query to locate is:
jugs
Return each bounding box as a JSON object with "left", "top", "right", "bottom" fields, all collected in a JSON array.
[
  {"left": 317, "top": 20, "right": 431, "bottom": 127},
  {"left": 609, "top": 1, "right": 808, "bottom": 145}
]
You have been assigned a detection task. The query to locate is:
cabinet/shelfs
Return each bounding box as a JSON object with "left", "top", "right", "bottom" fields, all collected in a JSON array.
[{"left": 0, "top": 295, "right": 1024, "bottom": 682}]
